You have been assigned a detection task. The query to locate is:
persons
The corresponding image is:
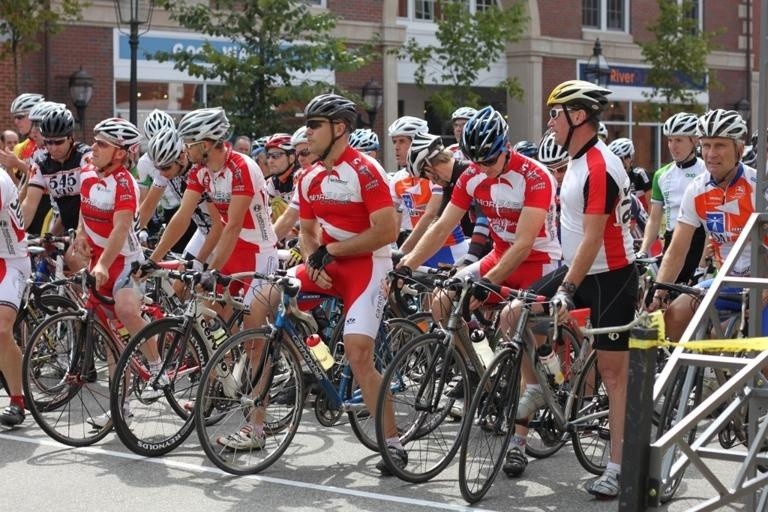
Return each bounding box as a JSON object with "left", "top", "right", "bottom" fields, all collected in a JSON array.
[{"left": 1, "top": 81, "right": 767, "bottom": 502}]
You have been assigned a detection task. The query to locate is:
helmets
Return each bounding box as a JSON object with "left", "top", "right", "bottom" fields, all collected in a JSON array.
[
  {"left": 94, "top": 118, "right": 141, "bottom": 149},
  {"left": 251, "top": 126, "right": 308, "bottom": 157},
  {"left": 349, "top": 105, "right": 539, "bottom": 163},
  {"left": 597, "top": 123, "right": 634, "bottom": 157},
  {"left": 547, "top": 80, "right": 612, "bottom": 106},
  {"left": 663, "top": 109, "right": 758, "bottom": 167},
  {"left": 539, "top": 127, "right": 568, "bottom": 166},
  {"left": 10, "top": 94, "right": 75, "bottom": 138},
  {"left": 144, "top": 106, "right": 230, "bottom": 168},
  {"left": 303, "top": 94, "right": 357, "bottom": 120}
]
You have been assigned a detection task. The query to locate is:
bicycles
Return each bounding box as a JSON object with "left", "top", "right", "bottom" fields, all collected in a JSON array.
[{"left": 1, "top": 230, "right": 768, "bottom": 501}]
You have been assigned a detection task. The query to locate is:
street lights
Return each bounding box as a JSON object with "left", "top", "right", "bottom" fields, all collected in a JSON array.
[
  {"left": 586, "top": 39, "right": 611, "bottom": 93},
  {"left": 111, "top": 0, "right": 151, "bottom": 126}
]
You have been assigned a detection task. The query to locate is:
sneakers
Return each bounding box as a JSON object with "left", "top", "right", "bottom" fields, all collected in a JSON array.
[
  {"left": 377, "top": 446, "right": 407, "bottom": 473},
  {"left": 217, "top": 425, "right": 265, "bottom": 450},
  {"left": 503, "top": 386, "right": 547, "bottom": 476},
  {"left": 0, "top": 406, "right": 25, "bottom": 425},
  {"left": 141, "top": 371, "right": 168, "bottom": 399},
  {"left": 87, "top": 409, "right": 133, "bottom": 428},
  {"left": 278, "top": 376, "right": 308, "bottom": 404},
  {"left": 444, "top": 366, "right": 485, "bottom": 396},
  {"left": 589, "top": 469, "right": 620, "bottom": 497}
]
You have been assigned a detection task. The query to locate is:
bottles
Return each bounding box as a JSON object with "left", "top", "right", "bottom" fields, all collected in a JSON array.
[
  {"left": 537, "top": 340, "right": 565, "bottom": 386},
  {"left": 305, "top": 334, "right": 337, "bottom": 372},
  {"left": 115, "top": 318, "right": 133, "bottom": 348},
  {"left": 208, "top": 318, "right": 232, "bottom": 358},
  {"left": 33, "top": 259, "right": 51, "bottom": 287},
  {"left": 469, "top": 327, "right": 495, "bottom": 368}
]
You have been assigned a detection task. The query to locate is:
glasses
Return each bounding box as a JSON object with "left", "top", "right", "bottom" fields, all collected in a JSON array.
[
  {"left": 307, "top": 121, "right": 329, "bottom": 128},
  {"left": 44, "top": 140, "right": 65, "bottom": 144},
  {"left": 93, "top": 138, "right": 119, "bottom": 150},
  {"left": 550, "top": 108, "right": 572, "bottom": 119}
]
